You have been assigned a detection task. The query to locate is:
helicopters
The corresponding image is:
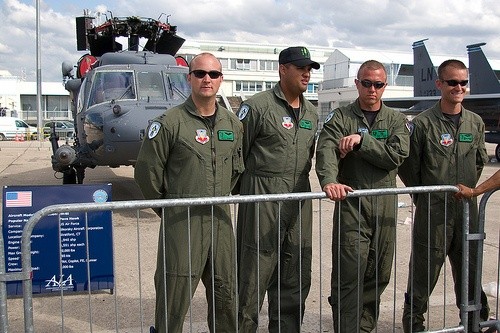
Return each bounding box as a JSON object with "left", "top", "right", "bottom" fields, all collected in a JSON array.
[{"left": 48, "top": 8, "right": 192, "bottom": 186}]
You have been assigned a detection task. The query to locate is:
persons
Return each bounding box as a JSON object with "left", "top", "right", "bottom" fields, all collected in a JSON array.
[
  {"left": 134, "top": 53, "right": 246, "bottom": 333},
  {"left": 453, "top": 168, "right": 500, "bottom": 201},
  {"left": 232, "top": 46, "right": 319, "bottom": 333},
  {"left": 398, "top": 59, "right": 489, "bottom": 333},
  {"left": 315, "top": 60, "right": 410, "bottom": 333}
]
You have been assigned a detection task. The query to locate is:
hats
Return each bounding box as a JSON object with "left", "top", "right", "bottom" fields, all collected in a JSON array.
[{"left": 279, "top": 47, "right": 320, "bottom": 69}]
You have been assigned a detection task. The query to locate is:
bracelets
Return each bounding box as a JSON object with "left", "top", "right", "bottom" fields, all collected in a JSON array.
[
  {"left": 470, "top": 188, "right": 473, "bottom": 200},
  {"left": 354, "top": 131, "right": 364, "bottom": 147}
]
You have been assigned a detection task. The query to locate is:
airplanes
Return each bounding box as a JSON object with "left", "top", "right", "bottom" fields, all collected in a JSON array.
[{"left": 381, "top": 37, "right": 500, "bottom": 164}]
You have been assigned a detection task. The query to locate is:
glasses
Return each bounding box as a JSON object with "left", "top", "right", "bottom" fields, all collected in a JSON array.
[
  {"left": 444, "top": 79, "right": 468, "bottom": 87},
  {"left": 357, "top": 79, "right": 386, "bottom": 89},
  {"left": 189, "top": 70, "right": 223, "bottom": 79}
]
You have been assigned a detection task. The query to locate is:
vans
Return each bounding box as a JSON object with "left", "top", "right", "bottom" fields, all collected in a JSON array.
[{"left": 0, "top": 116, "right": 37, "bottom": 141}]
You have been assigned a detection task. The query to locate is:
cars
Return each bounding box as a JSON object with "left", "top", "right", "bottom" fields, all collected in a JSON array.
[{"left": 44, "top": 121, "right": 76, "bottom": 139}]
